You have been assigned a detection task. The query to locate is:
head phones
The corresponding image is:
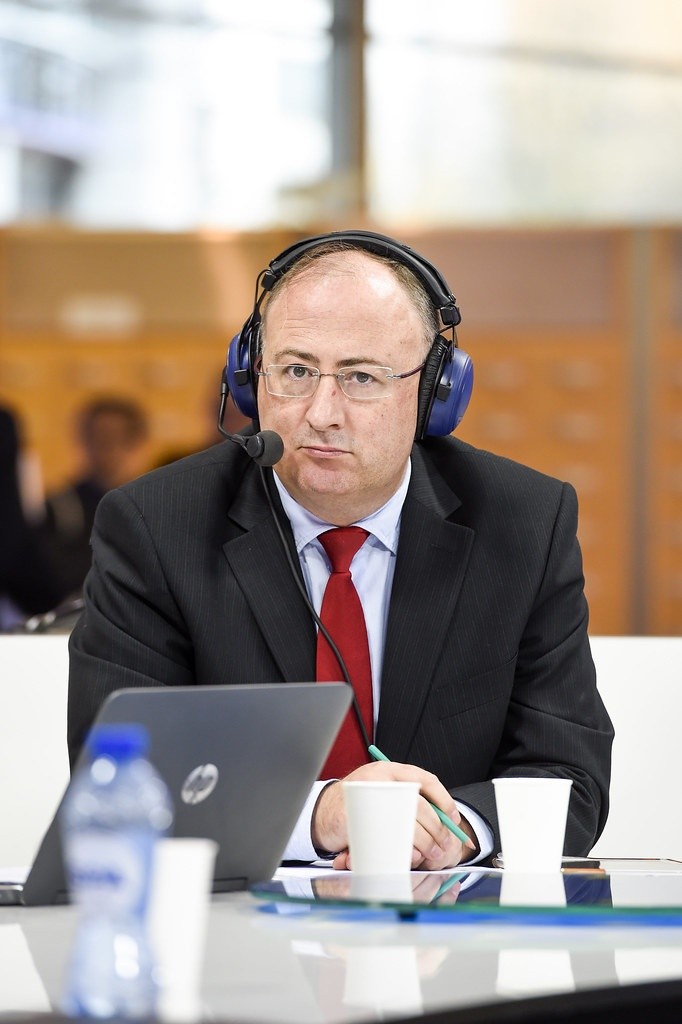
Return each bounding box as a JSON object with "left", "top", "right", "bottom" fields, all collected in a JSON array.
[{"left": 226, "top": 229, "right": 473, "bottom": 444}]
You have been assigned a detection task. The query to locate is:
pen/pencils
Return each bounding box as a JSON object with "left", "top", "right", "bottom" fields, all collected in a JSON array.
[
  {"left": 428, "top": 872, "right": 469, "bottom": 903},
  {"left": 368, "top": 744, "right": 476, "bottom": 851}
]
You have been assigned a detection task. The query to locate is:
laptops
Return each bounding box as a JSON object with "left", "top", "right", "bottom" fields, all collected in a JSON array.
[{"left": 0, "top": 681, "right": 354, "bottom": 907}]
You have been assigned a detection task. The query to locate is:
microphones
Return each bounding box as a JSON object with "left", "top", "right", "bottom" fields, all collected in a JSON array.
[{"left": 218, "top": 366, "right": 284, "bottom": 466}]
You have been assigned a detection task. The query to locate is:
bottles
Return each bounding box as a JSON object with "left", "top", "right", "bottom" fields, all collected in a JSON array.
[{"left": 57, "top": 723, "right": 173, "bottom": 1024}]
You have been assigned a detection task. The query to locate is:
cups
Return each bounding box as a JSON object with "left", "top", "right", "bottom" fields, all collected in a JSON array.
[
  {"left": 343, "top": 782, "right": 420, "bottom": 872},
  {"left": 153, "top": 839, "right": 221, "bottom": 967},
  {"left": 492, "top": 778, "right": 573, "bottom": 871}
]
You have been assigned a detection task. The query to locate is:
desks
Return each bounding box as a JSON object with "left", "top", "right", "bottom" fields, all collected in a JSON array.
[{"left": 0, "top": 883, "right": 682, "bottom": 1024}]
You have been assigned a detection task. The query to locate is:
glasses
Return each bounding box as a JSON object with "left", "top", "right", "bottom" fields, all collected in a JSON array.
[{"left": 254, "top": 352, "right": 425, "bottom": 400}]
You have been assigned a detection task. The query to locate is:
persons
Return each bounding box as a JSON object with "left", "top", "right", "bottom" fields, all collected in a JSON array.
[
  {"left": 66, "top": 228, "right": 616, "bottom": 868},
  {"left": 0, "top": 376, "right": 253, "bottom": 633},
  {"left": 255, "top": 868, "right": 620, "bottom": 1023}
]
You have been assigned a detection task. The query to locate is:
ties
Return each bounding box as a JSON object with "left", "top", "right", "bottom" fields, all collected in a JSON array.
[{"left": 315, "top": 526, "right": 373, "bottom": 780}]
